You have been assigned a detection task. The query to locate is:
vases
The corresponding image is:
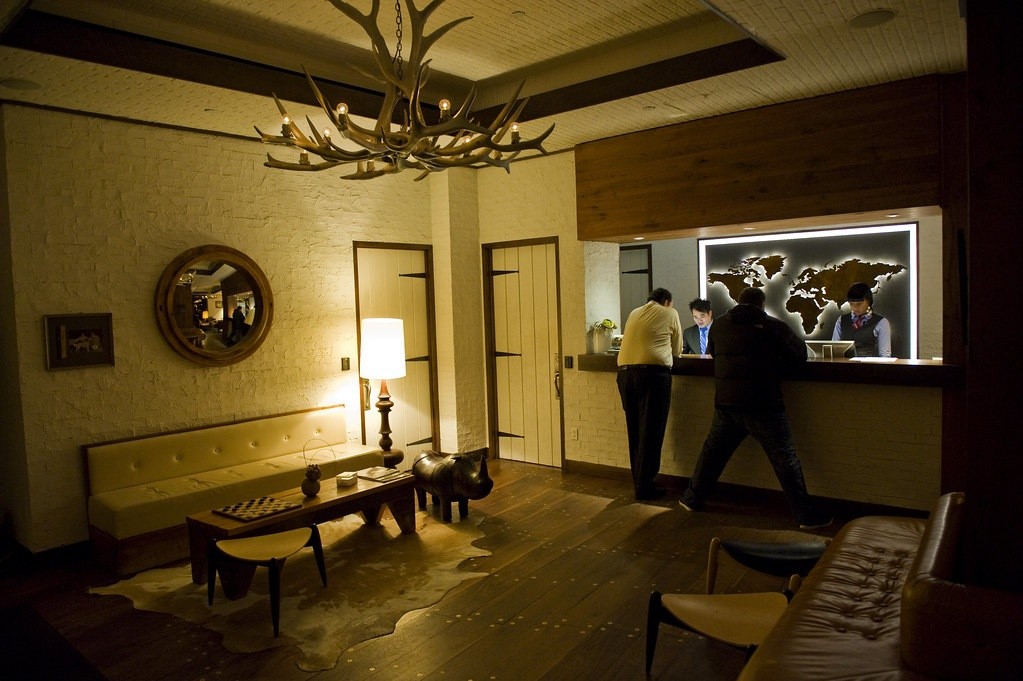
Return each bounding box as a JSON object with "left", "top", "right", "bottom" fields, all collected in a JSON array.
[
  {"left": 594, "top": 331, "right": 613, "bottom": 353},
  {"left": 301, "top": 479, "right": 321, "bottom": 497}
]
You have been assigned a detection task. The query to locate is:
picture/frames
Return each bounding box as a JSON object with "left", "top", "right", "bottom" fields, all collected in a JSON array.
[{"left": 43, "top": 313, "right": 117, "bottom": 372}]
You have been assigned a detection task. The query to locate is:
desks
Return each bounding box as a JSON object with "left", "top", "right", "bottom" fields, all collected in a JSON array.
[{"left": 186, "top": 466, "right": 418, "bottom": 586}]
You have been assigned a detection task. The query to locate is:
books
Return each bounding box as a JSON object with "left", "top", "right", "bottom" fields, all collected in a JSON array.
[{"left": 354, "top": 465, "right": 409, "bottom": 482}]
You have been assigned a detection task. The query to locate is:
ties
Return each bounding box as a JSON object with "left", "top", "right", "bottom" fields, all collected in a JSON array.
[{"left": 699, "top": 327, "right": 708, "bottom": 354}]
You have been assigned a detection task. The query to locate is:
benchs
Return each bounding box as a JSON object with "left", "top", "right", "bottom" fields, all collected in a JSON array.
[{"left": 81, "top": 403, "right": 384, "bottom": 579}]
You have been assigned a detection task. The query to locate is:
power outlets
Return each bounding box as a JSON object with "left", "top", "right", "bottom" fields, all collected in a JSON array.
[
  {"left": 342, "top": 357, "right": 350, "bottom": 371},
  {"left": 565, "top": 356, "right": 572, "bottom": 368},
  {"left": 571, "top": 428, "right": 577, "bottom": 440}
]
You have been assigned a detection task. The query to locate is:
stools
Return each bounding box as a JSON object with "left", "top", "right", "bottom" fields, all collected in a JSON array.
[
  {"left": 209, "top": 523, "right": 327, "bottom": 636},
  {"left": 706, "top": 534, "right": 831, "bottom": 593},
  {"left": 645, "top": 588, "right": 788, "bottom": 674}
]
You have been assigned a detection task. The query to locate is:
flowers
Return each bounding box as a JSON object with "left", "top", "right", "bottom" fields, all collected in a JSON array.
[
  {"left": 592, "top": 319, "right": 617, "bottom": 332},
  {"left": 303, "top": 439, "right": 337, "bottom": 481}
]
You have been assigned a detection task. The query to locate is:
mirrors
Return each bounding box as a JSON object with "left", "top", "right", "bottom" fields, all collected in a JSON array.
[{"left": 155, "top": 244, "right": 274, "bottom": 366}]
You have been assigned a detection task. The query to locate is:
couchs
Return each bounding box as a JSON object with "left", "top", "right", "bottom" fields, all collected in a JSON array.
[{"left": 735, "top": 491, "right": 1022, "bottom": 681}]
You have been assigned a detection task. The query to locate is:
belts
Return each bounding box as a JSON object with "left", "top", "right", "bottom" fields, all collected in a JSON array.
[{"left": 618, "top": 364, "right": 672, "bottom": 370}]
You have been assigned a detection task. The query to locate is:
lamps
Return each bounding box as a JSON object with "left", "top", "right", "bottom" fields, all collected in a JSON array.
[
  {"left": 253, "top": 0, "right": 556, "bottom": 185},
  {"left": 360, "top": 315, "right": 408, "bottom": 469}
]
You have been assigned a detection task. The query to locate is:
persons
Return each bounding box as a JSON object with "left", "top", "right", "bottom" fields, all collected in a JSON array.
[
  {"left": 829, "top": 283, "right": 893, "bottom": 358},
  {"left": 679, "top": 287, "right": 835, "bottom": 529},
  {"left": 680, "top": 297, "right": 717, "bottom": 353},
  {"left": 225, "top": 306, "right": 250, "bottom": 346},
  {"left": 615, "top": 286, "right": 685, "bottom": 502}
]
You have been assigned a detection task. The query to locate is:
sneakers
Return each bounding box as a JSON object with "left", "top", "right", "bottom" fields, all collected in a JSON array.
[
  {"left": 635, "top": 486, "right": 666, "bottom": 501},
  {"left": 800, "top": 514, "right": 833, "bottom": 530},
  {"left": 679, "top": 497, "right": 696, "bottom": 511}
]
